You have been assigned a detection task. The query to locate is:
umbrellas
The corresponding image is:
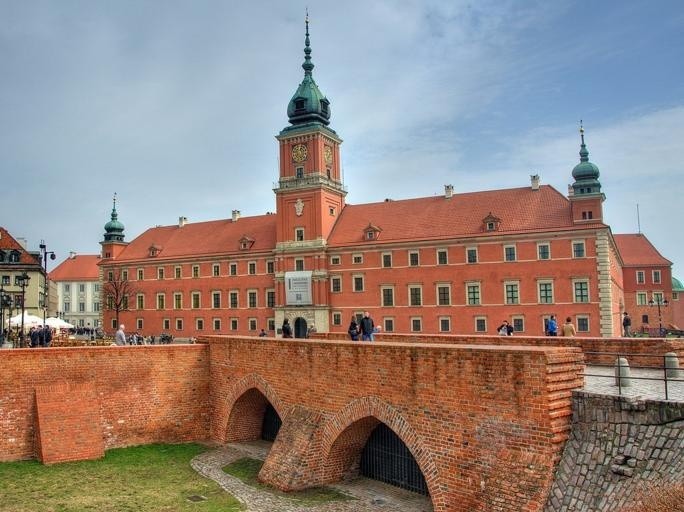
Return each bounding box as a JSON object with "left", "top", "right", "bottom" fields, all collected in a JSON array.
[{"left": 5, "top": 312, "right": 75, "bottom": 330}]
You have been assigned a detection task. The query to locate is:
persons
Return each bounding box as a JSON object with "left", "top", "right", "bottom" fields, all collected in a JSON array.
[
  {"left": 282, "top": 318, "right": 293, "bottom": 338},
  {"left": 4, "top": 324, "right": 54, "bottom": 348},
  {"left": 307, "top": 326, "right": 318, "bottom": 338},
  {"left": 115, "top": 324, "right": 175, "bottom": 346},
  {"left": 68, "top": 325, "right": 98, "bottom": 336},
  {"left": 561, "top": 317, "right": 576, "bottom": 337},
  {"left": 623, "top": 311, "right": 633, "bottom": 337},
  {"left": 348, "top": 315, "right": 360, "bottom": 340},
  {"left": 497, "top": 319, "right": 514, "bottom": 336},
  {"left": 259, "top": 328, "right": 267, "bottom": 337},
  {"left": 359, "top": 310, "right": 375, "bottom": 341},
  {"left": 548, "top": 314, "right": 557, "bottom": 336}
]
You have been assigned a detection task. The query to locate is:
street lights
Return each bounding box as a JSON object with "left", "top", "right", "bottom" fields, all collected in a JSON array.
[
  {"left": 648, "top": 297, "right": 669, "bottom": 337},
  {"left": 0, "top": 287, "right": 12, "bottom": 345},
  {"left": 39, "top": 240, "right": 56, "bottom": 346},
  {"left": 18, "top": 272, "right": 31, "bottom": 346}
]
